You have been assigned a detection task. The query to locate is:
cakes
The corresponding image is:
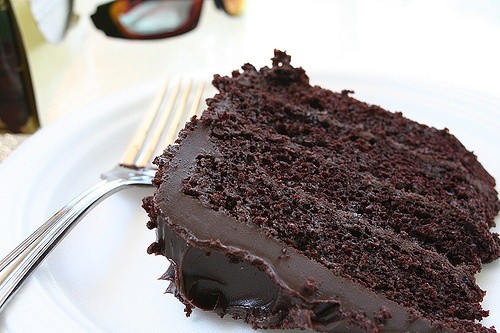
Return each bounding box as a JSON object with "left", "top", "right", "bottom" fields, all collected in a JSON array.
[{"left": 141, "top": 47, "right": 500, "bottom": 332}]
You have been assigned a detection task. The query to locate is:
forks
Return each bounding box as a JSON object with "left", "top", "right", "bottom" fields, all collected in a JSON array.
[{"left": 0, "top": 81, "right": 205, "bottom": 309}]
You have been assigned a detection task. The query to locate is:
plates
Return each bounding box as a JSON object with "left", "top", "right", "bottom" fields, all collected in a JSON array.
[{"left": 1, "top": 78, "right": 467, "bottom": 330}]
